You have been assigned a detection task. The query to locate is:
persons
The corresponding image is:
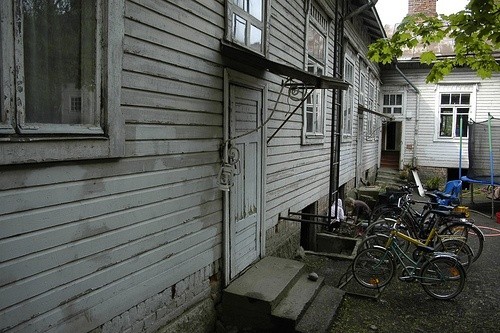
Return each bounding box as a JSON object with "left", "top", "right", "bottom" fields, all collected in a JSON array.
[
  {"left": 325, "top": 199, "right": 344, "bottom": 230},
  {"left": 344, "top": 197, "right": 371, "bottom": 226}
]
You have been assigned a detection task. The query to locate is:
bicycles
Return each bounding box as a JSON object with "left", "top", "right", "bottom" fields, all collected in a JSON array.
[
  {"left": 353, "top": 218, "right": 467, "bottom": 301},
  {"left": 357, "top": 184, "right": 485, "bottom": 279}
]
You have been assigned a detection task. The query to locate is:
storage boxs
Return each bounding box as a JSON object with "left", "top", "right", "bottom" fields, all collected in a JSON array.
[{"left": 358, "top": 186, "right": 381, "bottom": 199}]
